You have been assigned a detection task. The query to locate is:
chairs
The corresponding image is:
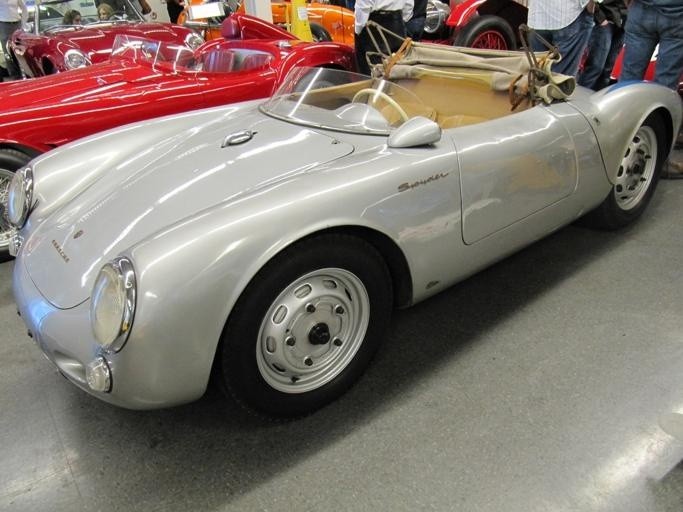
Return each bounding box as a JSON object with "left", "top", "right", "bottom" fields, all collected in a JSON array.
[
  {"left": 199, "top": 48, "right": 272, "bottom": 72},
  {"left": 382, "top": 99, "right": 489, "bottom": 133}
]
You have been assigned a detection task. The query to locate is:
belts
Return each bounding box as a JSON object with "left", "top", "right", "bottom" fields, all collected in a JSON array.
[{"left": 375, "top": 10, "right": 403, "bottom": 15}]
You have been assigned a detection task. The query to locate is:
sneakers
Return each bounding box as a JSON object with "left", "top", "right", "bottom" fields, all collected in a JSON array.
[{"left": 661, "top": 159, "right": 683, "bottom": 179}]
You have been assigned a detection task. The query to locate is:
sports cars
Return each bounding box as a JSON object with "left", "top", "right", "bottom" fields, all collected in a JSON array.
[
  {"left": 4, "top": 0, "right": 207, "bottom": 82},
  {"left": 9, "top": 34, "right": 683, "bottom": 415},
  {"left": 0, "top": 29, "right": 363, "bottom": 265}
]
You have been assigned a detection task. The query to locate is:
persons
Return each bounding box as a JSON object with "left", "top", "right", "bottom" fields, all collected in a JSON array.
[
  {"left": 166, "top": 0, "right": 186, "bottom": 25},
  {"left": 354, "top": 0, "right": 416, "bottom": 77},
  {"left": 403, "top": 0, "right": 427, "bottom": 40},
  {"left": 526, "top": 0, "right": 682, "bottom": 180},
  {"left": 98, "top": 3, "right": 117, "bottom": 22},
  {"left": 62, "top": 9, "right": 82, "bottom": 28},
  {"left": 0, "top": 0, "right": 29, "bottom": 69}
]
